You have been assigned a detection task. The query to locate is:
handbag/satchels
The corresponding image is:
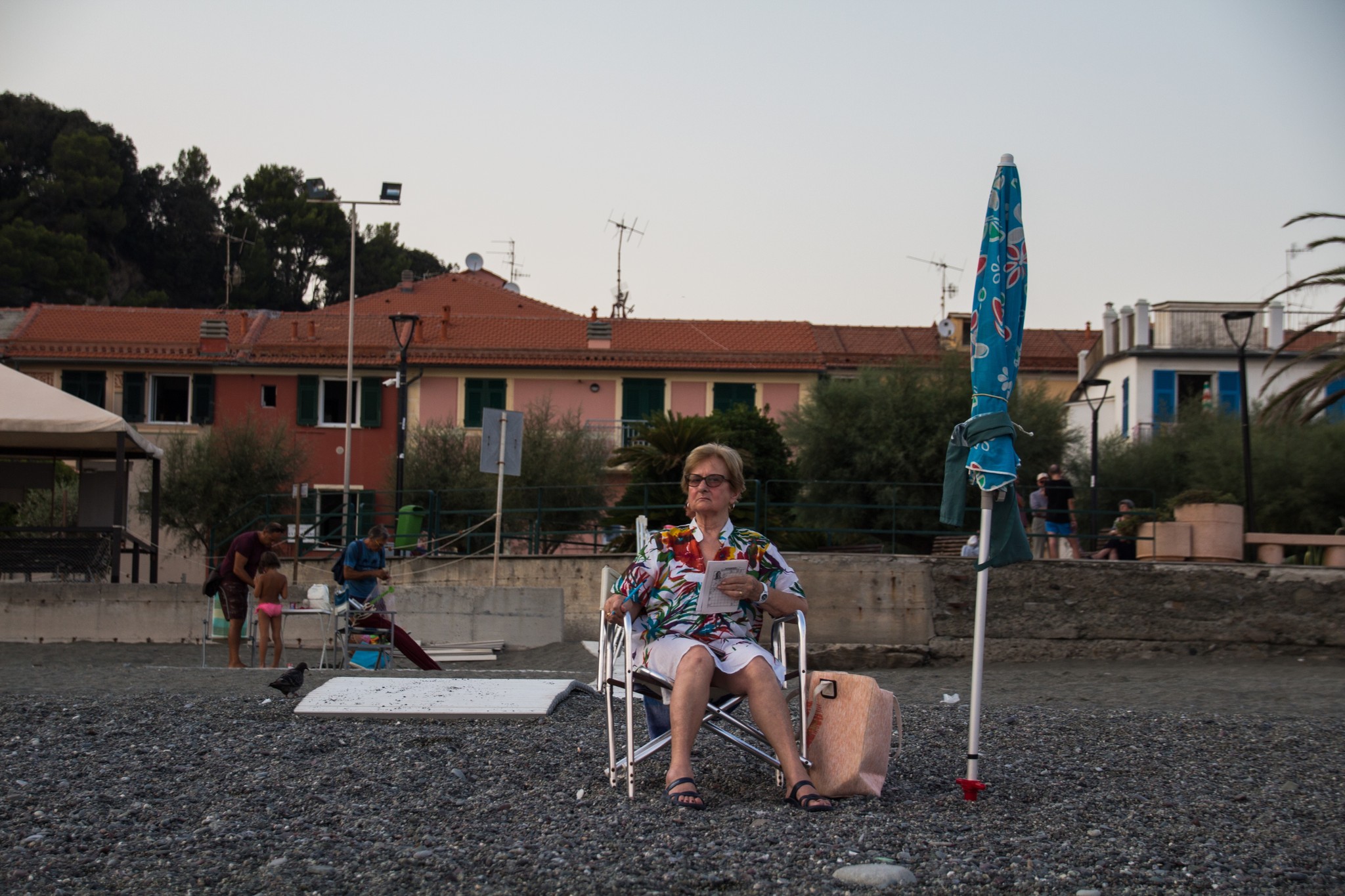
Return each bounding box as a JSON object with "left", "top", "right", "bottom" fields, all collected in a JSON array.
[
  {"left": 784, "top": 671, "right": 903, "bottom": 799},
  {"left": 1059, "top": 536, "right": 1081, "bottom": 561},
  {"left": 201, "top": 566, "right": 224, "bottom": 597}
]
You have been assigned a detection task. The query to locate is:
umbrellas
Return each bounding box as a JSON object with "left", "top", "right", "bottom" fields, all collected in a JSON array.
[
  {"left": 956, "top": 153, "right": 1027, "bottom": 802},
  {"left": 347, "top": 594, "right": 442, "bottom": 671}
]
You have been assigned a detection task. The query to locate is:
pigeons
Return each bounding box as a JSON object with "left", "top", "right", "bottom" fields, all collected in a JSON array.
[{"left": 268, "top": 662, "right": 310, "bottom": 698}]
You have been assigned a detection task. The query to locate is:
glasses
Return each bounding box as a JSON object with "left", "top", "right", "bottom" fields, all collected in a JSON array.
[
  {"left": 1038, "top": 480, "right": 1045, "bottom": 484},
  {"left": 683, "top": 473, "right": 735, "bottom": 488},
  {"left": 375, "top": 539, "right": 384, "bottom": 549}
]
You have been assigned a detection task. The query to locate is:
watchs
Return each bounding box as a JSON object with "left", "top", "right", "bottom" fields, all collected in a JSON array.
[{"left": 753, "top": 581, "right": 768, "bottom": 604}]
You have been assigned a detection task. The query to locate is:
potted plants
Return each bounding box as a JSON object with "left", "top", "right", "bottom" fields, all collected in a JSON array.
[
  {"left": 1112, "top": 505, "right": 1194, "bottom": 565},
  {"left": 1163, "top": 487, "right": 1247, "bottom": 564}
]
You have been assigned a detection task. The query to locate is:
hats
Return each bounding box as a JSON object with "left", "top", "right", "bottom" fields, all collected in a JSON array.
[
  {"left": 1036, "top": 472, "right": 1049, "bottom": 481},
  {"left": 968, "top": 534, "right": 977, "bottom": 545},
  {"left": 1119, "top": 499, "right": 1134, "bottom": 508}
]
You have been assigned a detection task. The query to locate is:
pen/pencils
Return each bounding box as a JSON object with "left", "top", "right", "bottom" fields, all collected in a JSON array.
[{"left": 608, "top": 579, "right": 647, "bottom": 618}]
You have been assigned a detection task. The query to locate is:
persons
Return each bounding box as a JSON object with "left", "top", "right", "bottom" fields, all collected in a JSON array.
[
  {"left": 1029, "top": 473, "right": 1049, "bottom": 560},
  {"left": 253, "top": 552, "right": 288, "bottom": 667},
  {"left": 343, "top": 523, "right": 391, "bottom": 656},
  {"left": 1083, "top": 499, "right": 1136, "bottom": 560},
  {"left": 1013, "top": 472, "right": 1025, "bottom": 526},
  {"left": 216, "top": 522, "right": 284, "bottom": 668},
  {"left": 1040, "top": 464, "right": 1081, "bottom": 560},
  {"left": 603, "top": 440, "right": 836, "bottom": 812}
]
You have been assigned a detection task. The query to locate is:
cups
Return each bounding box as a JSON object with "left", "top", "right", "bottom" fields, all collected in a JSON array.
[
  {"left": 303, "top": 599, "right": 309, "bottom": 609},
  {"left": 290, "top": 602, "right": 296, "bottom": 609}
]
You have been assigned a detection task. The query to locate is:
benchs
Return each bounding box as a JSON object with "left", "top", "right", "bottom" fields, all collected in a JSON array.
[
  {"left": 812, "top": 542, "right": 887, "bottom": 554},
  {"left": 929, "top": 535, "right": 971, "bottom": 557},
  {"left": 1240, "top": 531, "right": 1345, "bottom": 569}
]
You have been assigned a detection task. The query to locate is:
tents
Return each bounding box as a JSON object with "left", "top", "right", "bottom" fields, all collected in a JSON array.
[{"left": 0, "top": 362, "right": 165, "bottom": 588}]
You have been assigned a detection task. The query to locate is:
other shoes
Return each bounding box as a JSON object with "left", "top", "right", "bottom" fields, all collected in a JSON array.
[{"left": 1085, "top": 554, "right": 1093, "bottom": 560}]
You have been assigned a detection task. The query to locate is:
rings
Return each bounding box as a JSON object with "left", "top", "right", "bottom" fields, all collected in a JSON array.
[
  {"left": 739, "top": 591, "right": 742, "bottom": 597},
  {"left": 605, "top": 612, "right": 611, "bottom": 617}
]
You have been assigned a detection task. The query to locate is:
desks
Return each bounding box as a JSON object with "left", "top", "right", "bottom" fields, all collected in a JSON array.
[{"left": 274, "top": 607, "right": 335, "bottom": 670}]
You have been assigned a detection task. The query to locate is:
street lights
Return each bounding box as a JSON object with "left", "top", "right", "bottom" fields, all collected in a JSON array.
[
  {"left": 388, "top": 311, "right": 423, "bottom": 556},
  {"left": 1221, "top": 310, "right": 1261, "bottom": 558},
  {"left": 302, "top": 178, "right": 403, "bottom": 551},
  {"left": 1080, "top": 377, "right": 1113, "bottom": 549}
]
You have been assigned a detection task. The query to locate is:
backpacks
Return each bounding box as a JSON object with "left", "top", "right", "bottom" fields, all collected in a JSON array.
[
  {"left": 331, "top": 540, "right": 383, "bottom": 586},
  {"left": 307, "top": 583, "right": 334, "bottom": 612}
]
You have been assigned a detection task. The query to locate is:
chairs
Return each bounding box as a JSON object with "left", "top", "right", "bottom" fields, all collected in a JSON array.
[
  {"left": 331, "top": 584, "right": 399, "bottom": 673},
  {"left": 200, "top": 588, "right": 259, "bottom": 670},
  {"left": 599, "top": 512, "right": 815, "bottom": 806}
]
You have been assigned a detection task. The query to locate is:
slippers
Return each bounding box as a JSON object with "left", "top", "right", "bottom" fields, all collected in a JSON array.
[
  {"left": 660, "top": 776, "right": 707, "bottom": 810},
  {"left": 783, "top": 779, "right": 834, "bottom": 812}
]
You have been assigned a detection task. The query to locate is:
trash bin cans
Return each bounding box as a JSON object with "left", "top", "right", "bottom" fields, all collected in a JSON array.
[{"left": 395, "top": 505, "right": 423, "bottom": 550}]
